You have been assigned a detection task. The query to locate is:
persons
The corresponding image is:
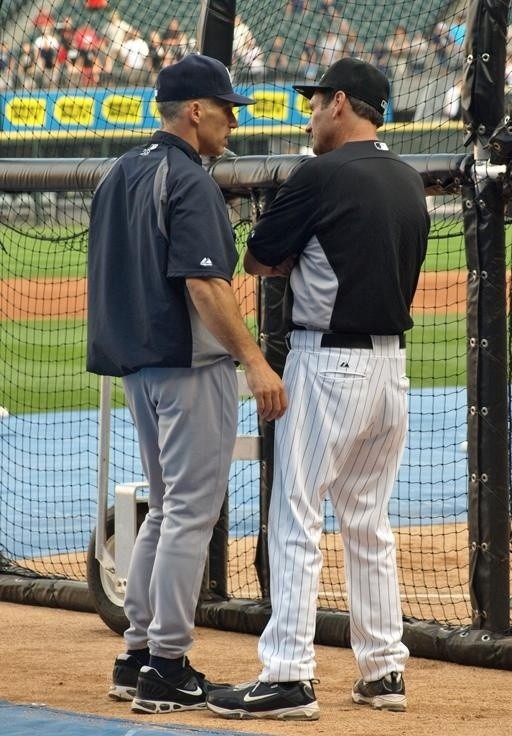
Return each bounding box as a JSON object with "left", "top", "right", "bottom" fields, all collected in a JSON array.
[
  {"left": 207, "top": 58, "right": 431, "bottom": 722},
  {"left": 86, "top": 55, "right": 289, "bottom": 711}
]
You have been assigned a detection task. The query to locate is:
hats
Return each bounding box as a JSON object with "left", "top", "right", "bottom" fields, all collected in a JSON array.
[
  {"left": 155, "top": 53, "right": 258, "bottom": 106},
  {"left": 292, "top": 57, "right": 391, "bottom": 117}
]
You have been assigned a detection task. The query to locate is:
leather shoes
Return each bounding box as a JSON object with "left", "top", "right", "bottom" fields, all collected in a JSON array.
[{"left": 321, "top": 331, "right": 407, "bottom": 350}]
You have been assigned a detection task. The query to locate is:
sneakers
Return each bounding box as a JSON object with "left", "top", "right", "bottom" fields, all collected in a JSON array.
[
  {"left": 107, "top": 652, "right": 148, "bottom": 701},
  {"left": 131, "top": 657, "right": 232, "bottom": 714},
  {"left": 351, "top": 671, "right": 406, "bottom": 711},
  {"left": 207, "top": 679, "right": 321, "bottom": 720}
]
